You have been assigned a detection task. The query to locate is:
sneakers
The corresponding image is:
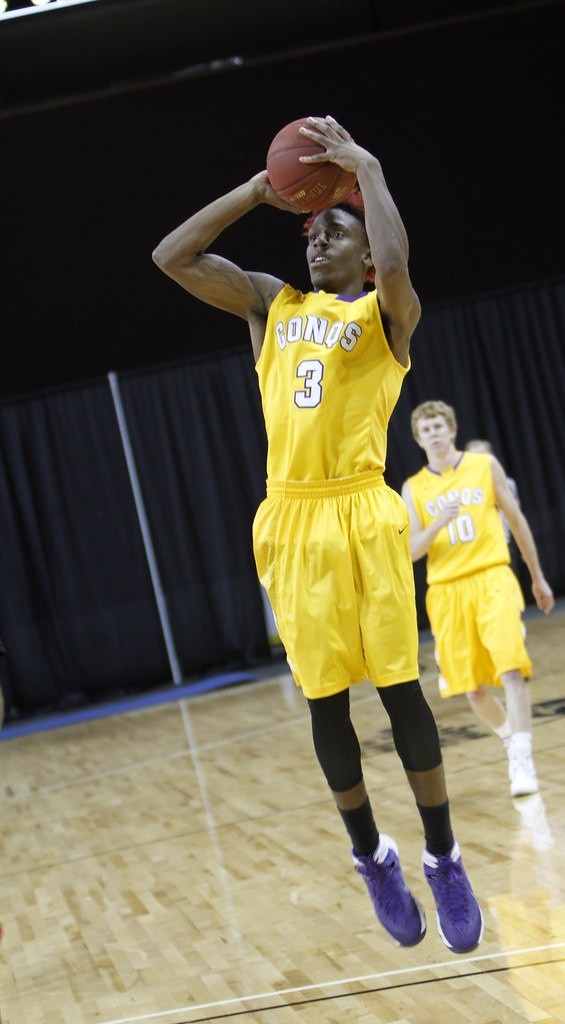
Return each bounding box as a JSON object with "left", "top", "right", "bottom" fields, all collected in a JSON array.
[
  {"left": 423, "top": 839, "right": 481, "bottom": 953},
  {"left": 507, "top": 733, "right": 538, "bottom": 796},
  {"left": 352, "top": 833, "right": 427, "bottom": 946}
]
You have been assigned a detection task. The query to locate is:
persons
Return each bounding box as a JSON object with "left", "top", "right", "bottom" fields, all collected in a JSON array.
[
  {"left": 465, "top": 440, "right": 518, "bottom": 547},
  {"left": 151, "top": 115, "right": 482, "bottom": 956},
  {"left": 402, "top": 401, "right": 554, "bottom": 795}
]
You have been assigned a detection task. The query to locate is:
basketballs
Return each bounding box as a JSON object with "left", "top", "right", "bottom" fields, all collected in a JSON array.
[{"left": 266, "top": 116, "right": 357, "bottom": 214}]
